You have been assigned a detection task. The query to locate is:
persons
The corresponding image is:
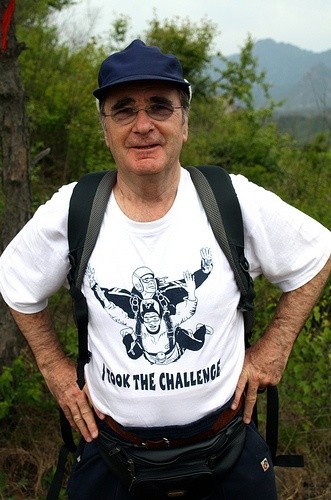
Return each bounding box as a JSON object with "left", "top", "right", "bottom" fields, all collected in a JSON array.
[{"left": 0, "top": 38, "right": 331, "bottom": 500}]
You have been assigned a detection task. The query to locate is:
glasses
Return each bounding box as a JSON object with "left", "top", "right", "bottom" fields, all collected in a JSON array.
[{"left": 102, "top": 101, "right": 185, "bottom": 125}]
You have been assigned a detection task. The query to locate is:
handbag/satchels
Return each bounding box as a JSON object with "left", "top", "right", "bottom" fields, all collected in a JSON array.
[{"left": 98, "top": 417, "right": 248, "bottom": 500}]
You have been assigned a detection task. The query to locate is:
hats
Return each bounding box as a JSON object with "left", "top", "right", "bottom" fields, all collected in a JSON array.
[{"left": 91, "top": 38, "right": 192, "bottom": 113}]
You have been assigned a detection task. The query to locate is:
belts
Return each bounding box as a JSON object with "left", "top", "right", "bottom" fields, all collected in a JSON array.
[{"left": 104, "top": 399, "right": 240, "bottom": 450}]
